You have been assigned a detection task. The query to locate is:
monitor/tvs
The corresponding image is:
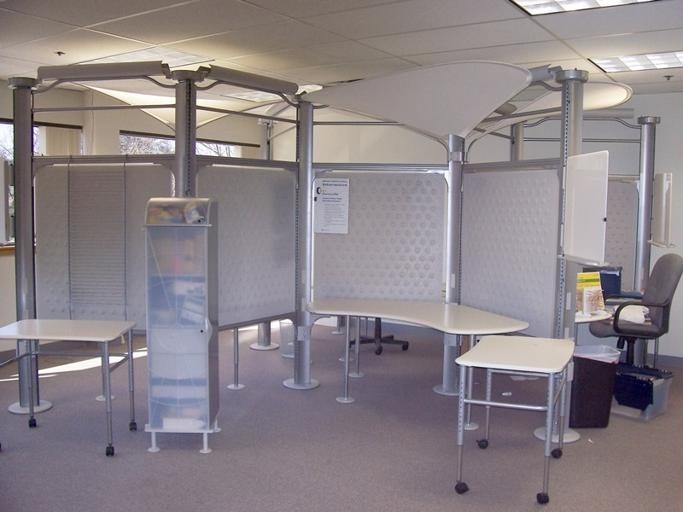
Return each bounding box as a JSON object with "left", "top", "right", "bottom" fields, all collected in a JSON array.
[{"left": 583, "top": 265, "right": 622, "bottom": 296}]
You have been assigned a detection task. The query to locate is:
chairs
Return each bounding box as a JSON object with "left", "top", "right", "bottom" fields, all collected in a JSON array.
[{"left": 589, "top": 253, "right": 683, "bottom": 377}]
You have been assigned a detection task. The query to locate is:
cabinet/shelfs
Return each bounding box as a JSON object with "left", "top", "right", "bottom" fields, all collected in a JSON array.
[{"left": 144, "top": 196, "right": 219, "bottom": 454}]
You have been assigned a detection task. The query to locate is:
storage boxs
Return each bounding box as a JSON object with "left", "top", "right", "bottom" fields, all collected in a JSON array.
[{"left": 610, "top": 362, "right": 675, "bottom": 422}]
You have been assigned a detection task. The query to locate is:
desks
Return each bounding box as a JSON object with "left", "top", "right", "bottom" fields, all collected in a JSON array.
[
  {"left": 1, "top": 319, "right": 137, "bottom": 456},
  {"left": 305, "top": 300, "right": 530, "bottom": 430},
  {"left": 455, "top": 334, "right": 578, "bottom": 504}
]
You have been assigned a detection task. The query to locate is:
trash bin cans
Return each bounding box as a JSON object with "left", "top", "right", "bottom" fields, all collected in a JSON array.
[{"left": 568, "top": 344, "right": 621, "bottom": 428}]
